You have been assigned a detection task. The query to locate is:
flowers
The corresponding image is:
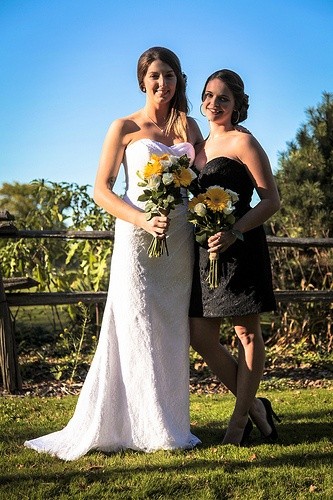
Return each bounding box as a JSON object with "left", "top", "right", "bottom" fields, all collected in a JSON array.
[
  {"left": 187, "top": 184, "right": 239, "bottom": 290},
  {"left": 134, "top": 151, "right": 192, "bottom": 259}
]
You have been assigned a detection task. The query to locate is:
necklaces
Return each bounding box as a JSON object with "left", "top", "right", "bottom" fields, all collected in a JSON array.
[{"left": 143, "top": 108, "right": 174, "bottom": 132}]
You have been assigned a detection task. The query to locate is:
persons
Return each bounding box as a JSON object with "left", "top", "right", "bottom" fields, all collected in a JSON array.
[
  {"left": 189, "top": 69, "right": 280, "bottom": 445},
  {"left": 93, "top": 47, "right": 205, "bottom": 453}
]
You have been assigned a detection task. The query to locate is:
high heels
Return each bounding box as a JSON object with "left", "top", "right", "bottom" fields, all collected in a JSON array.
[
  {"left": 220, "top": 417, "right": 253, "bottom": 447},
  {"left": 257, "top": 398, "right": 282, "bottom": 444}
]
